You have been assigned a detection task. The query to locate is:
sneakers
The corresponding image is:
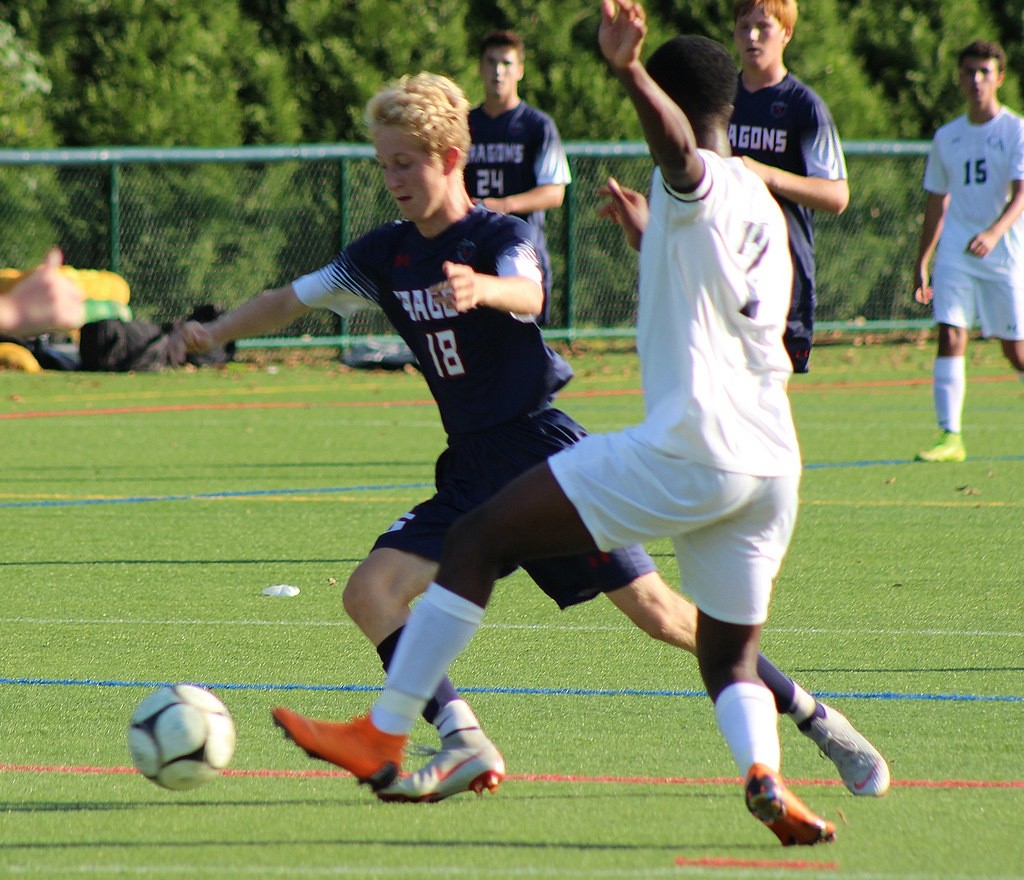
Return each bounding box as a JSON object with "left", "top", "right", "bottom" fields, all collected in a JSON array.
[
  {"left": 801, "top": 699, "right": 890, "bottom": 797},
  {"left": 272, "top": 708, "right": 408, "bottom": 793},
  {"left": 743, "top": 763, "right": 836, "bottom": 845},
  {"left": 377, "top": 742, "right": 506, "bottom": 803},
  {"left": 915, "top": 434, "right": 966, "bottom": 462}
]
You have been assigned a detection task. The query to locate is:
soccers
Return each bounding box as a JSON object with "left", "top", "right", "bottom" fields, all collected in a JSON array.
[{"left": 125, "top": 683, "right": 238, "bottom": 791}]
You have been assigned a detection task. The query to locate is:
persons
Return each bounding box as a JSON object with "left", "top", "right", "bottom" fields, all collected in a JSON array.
[
  {"left": 465, "top": 31, "right": 574, "bottom": 243},
  {"left": 270, "top": 0, "right": 836, "bottom": 845},
  {"left": 728, "top": 0, "right": 850, "bottom": 373},
  {"left": 168, "top": 70, "right": 891, "bottom": 799},
  {"left": 916, "top": 41, "right": 1024, "bottom": 462}
]
